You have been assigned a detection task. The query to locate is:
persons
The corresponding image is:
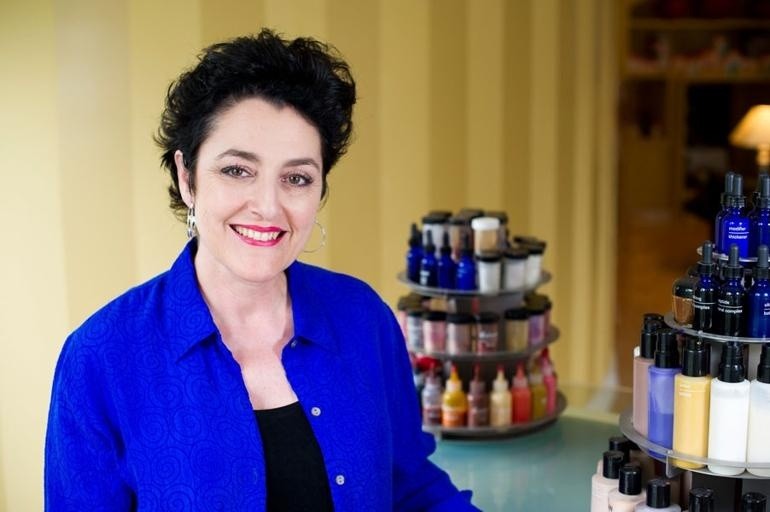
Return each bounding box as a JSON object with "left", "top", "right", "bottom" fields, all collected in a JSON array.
[{"left": 43, "top": 25, "right": 481, "bottom": 509}]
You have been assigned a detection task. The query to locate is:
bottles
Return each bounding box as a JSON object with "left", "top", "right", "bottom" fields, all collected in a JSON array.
[
  {"left": 394, "top": 289, "right": 558, "bottom": 432},
  {"left": 409, "top": 207, "right": 546, "bottom": 295},
  {"left": 672, "top": 170, "right": 769, "bottom": 339},
  {"left": 589, "top": 310, "right": 770, "bottom": 512}
]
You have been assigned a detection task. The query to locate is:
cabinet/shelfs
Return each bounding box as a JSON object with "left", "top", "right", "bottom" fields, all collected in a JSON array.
[
  {"left": 387, "top": 266, "right": 568, "bottom": 442},
  {"left": 613, "top": 239, "right": 770, "bottom": 512}
]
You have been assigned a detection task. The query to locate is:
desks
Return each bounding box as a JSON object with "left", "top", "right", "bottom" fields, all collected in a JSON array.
[{"left": 406, "top": 409, "right": 621, "bottom": 512}]
